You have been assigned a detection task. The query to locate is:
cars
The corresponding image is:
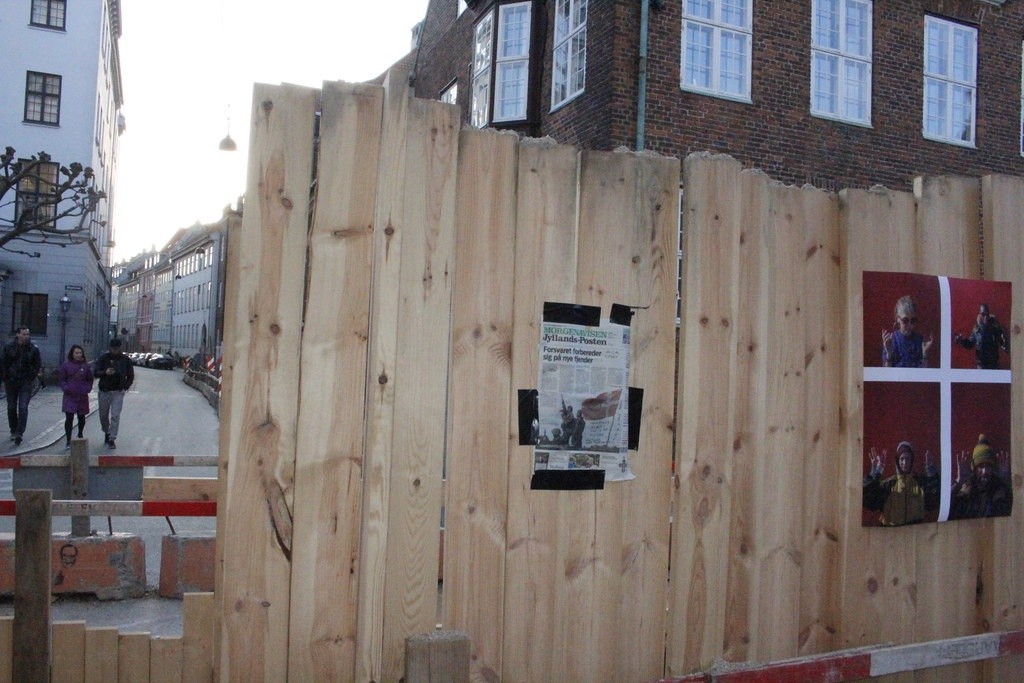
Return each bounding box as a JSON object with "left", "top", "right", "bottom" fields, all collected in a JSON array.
[
  {"left": 148, "top": 353, "right": 174, "bottom": 371},
  {"left": 123, "top": 352, "right": 153, "bottom": 367}
]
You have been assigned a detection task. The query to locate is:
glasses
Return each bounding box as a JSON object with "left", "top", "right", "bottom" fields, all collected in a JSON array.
[
  {"left": 898, "top": 314, "right": 917, "bottom": 324},
  {"left": 980, "top": 312, "right": 989, "bottom": 316}
]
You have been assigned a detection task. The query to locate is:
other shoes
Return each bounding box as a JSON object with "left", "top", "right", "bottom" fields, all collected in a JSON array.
[
  {"left": 105, "top": 433, "right": 110, "bottom": 443},
  {"left": 9, "top": 434, "right": 17, "bottom": 440},
  {"left": 109, "top": 440, "right": 116, "bottom": 449},
  {"left": 15, "top": 436, "right": 22, "bottom": 445},
  {"left": 77, "top": 433, "right": 83, "bottom": 438},
  {"left": 65, "top": 443, "right": 71, "bottom": 449}
]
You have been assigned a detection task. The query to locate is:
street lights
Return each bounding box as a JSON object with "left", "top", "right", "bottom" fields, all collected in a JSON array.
[{"left": 59, "top": 293, "right": 72, "bottom": 366}]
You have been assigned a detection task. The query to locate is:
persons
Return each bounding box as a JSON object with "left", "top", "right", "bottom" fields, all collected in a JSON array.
[
  {"left": 93, "top": 337, "right": 135, "bottom": 450},
  {"left": 949, "top": 433, "right": 1013, "bottom": 519},
  {"left": 2, "top": 324, "right": 42, "bottom": 447},
  {"left": 863, "top": 440, "right": 942, "bottom": 527},
  {"left": 543, "top": 404, "right": 587, "bottom": 449},
  {"left": 58, "top": 344, "right": 94, "bottom": 451},
  {"left": 954, "top": 302, "right": 1010, "bottom": 369},
  {"left": 881, "top": 294, "right": 936, "bottom": 369}
]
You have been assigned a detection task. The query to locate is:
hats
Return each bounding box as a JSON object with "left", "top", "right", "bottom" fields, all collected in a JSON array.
[
  {"left": 110, "top": 339, "right": 122, "bottom": 347},
  {"left": 971, "top": 434, "right": 997, "bottom": 468},
  {"left": 895, "top": 441, "right": 914, "bottom": 460}
]
